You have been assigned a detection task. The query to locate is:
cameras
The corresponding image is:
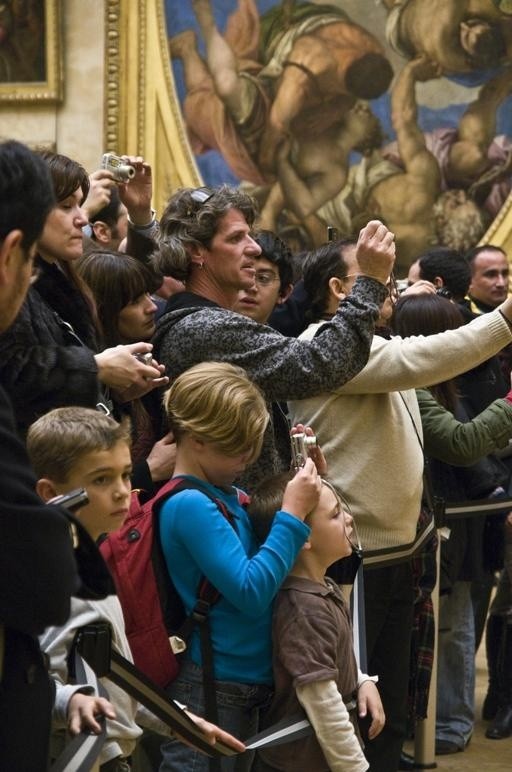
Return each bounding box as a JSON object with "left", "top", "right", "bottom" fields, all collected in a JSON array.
[
  {"left": 290, "top": 433, "right": 317, "bottom": 471},
  {"left": 132, "top": 351, "right": 153, "bottom": 365},
  {"left": 437, "top": 288, "right": 452, "bottom": 297},
  {"left": 47, "top": 486, "right": 90, "bottom": 510},
  {"left": 103, "top": 153, "right": 135, "bottom": 183}
]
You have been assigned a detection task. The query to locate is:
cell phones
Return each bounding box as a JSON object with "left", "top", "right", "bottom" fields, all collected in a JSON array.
[{"left": 328, "top": 227, "right": 337, "bottom": 240}]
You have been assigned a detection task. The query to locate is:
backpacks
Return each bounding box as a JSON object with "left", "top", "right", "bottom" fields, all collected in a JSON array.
[{"left": 95, "top": 476, "right": 240, "bottom": 688}]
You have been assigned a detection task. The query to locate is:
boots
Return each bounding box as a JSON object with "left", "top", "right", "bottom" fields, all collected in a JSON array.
[{"left": 482, "top": 617, "right": 511, "bottom": 739}]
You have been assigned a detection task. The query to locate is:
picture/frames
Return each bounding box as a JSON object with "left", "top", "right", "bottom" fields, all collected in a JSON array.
[{"left": 1, "top": 0, "right": 66, "bottom": 103}]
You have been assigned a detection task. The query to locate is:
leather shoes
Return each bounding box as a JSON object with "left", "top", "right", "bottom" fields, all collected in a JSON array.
[
  {"left": 434, "top": 737, "right": 459, "bottom": 754},
  {"left": 398, "top": 750, "right": 435, "bottom": 770}
]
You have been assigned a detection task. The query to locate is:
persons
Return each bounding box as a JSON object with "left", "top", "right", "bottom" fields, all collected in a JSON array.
[{"left": 0, "top": 138, "right": 512, "bottom": 772}]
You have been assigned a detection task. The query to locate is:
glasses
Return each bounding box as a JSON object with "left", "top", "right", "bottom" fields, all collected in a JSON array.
[{"left": 189, "top": 184, "right": 215, "bottom": 220}]
